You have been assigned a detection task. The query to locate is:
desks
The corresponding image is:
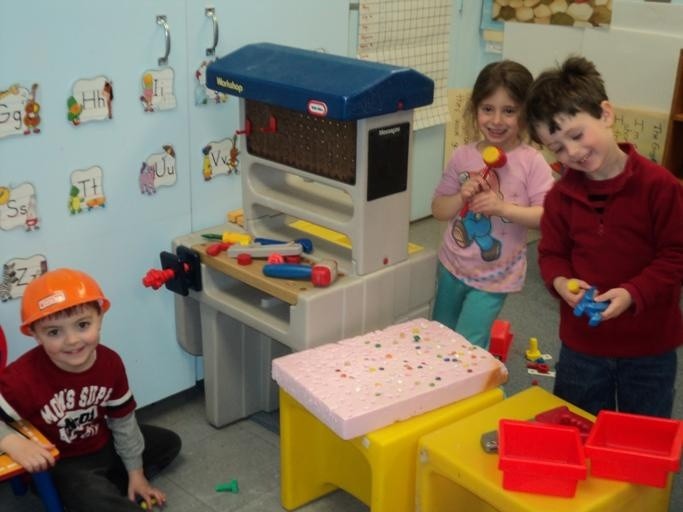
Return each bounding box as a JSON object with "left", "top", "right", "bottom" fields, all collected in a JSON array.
[{"left": 0, "top": 421, "right": 63, "bottom": 512}]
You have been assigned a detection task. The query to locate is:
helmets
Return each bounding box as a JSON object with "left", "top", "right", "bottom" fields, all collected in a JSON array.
[{"left": 16, "top": 268, "right": 112, "bottom": 335}]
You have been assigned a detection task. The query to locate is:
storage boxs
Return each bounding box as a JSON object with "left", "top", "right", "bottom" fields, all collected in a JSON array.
[
  {"left": 499, "top": 419, "right": 587, "bottom": 498},
  {"left": 583, "top": 409, "right": 683, "bottom": 488}
]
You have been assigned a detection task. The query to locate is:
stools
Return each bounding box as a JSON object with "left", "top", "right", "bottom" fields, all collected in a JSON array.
[
  {"left": 279, "top": 384, "right": 504, "bottom": 512},
  {"left": 415, "top": 385, "right": 674, "bottom": 512}
]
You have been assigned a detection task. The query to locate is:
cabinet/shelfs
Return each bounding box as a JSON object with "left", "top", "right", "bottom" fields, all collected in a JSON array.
[{"left": 662, "top": 48, "right": 683, "bottom": 179}]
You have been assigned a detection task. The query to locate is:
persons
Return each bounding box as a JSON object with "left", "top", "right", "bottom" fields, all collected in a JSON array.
[
  {"left": 428, "top": 61, "right": 554, "bottom": 347},
  {"left": 525, "top": 55, "right": 681, "bottom": 419},
  {"left": 0, "top": 269, "right": 181, "bottom": 512}
]
